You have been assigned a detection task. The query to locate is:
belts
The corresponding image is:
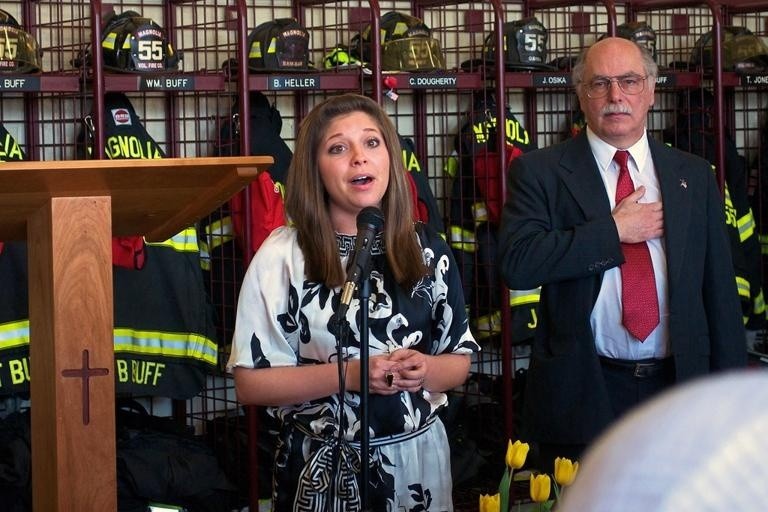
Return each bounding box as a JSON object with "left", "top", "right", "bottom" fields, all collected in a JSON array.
[{"left": 600, "top": 355, "right": 672, "bottom": 377}]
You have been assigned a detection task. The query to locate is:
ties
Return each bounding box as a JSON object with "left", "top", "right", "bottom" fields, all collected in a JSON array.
[{"left": 614, "top": 150, "right": 660, "bottom": 342}]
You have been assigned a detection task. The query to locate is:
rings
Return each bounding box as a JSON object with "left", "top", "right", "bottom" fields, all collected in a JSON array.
[
  {"left": 385, "top": 370, "right": 393, "bottom": 387},
  {"left": 419, "top": 377, "right": 425, "bottom": 386}
]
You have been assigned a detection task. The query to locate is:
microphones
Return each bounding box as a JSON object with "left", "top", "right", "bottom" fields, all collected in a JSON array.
[{"left": 337, "top": 207, "right": 386, "bottom": 324}]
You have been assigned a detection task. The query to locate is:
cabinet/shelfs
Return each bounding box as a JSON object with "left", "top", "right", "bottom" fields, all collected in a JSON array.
[{"left": 0, "top": 0, "right": 768, "bottom": 512}]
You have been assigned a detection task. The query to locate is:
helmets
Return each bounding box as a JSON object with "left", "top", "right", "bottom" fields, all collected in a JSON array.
[
  {"left": 483, "top": 17, "right": 559, "bottom": 71},
  {"left": 356, "top": 12, "right": 447, "bottom": 72},
  {"left": 86, "top": 11, "right": 183, "bottom": 75},
  {"left": 600, "top": 20, "right": 657, "bottom": 63},
  {"left": 0, "top": 9, "right": 42, "bottom": 74},
  {"left": 691, "top": 24, "right": 767, "bottom": 75},
  {"left": 245, "top": 17, "right": 318, "bottom": 73}
]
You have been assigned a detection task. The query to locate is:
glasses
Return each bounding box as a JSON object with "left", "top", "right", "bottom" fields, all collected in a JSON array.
[{"left": 579, "top": 71, "right": 652, "bottom": 98}]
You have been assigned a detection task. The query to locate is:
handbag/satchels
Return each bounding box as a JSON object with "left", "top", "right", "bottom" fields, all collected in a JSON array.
[
  {"left": 0, "top": 398, "right": 248, "bottom": 511},
  {"left": 204, "top": 402, "right": 276, "bottom": 500}
]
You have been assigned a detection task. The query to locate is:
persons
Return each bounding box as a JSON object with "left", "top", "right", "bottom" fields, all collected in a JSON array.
[
  {"left": 489, "top": 37, "right": 746, "bottom": 501},
  {"left": 225, "top": 90, "right": 483, "bottom": 512}
]
